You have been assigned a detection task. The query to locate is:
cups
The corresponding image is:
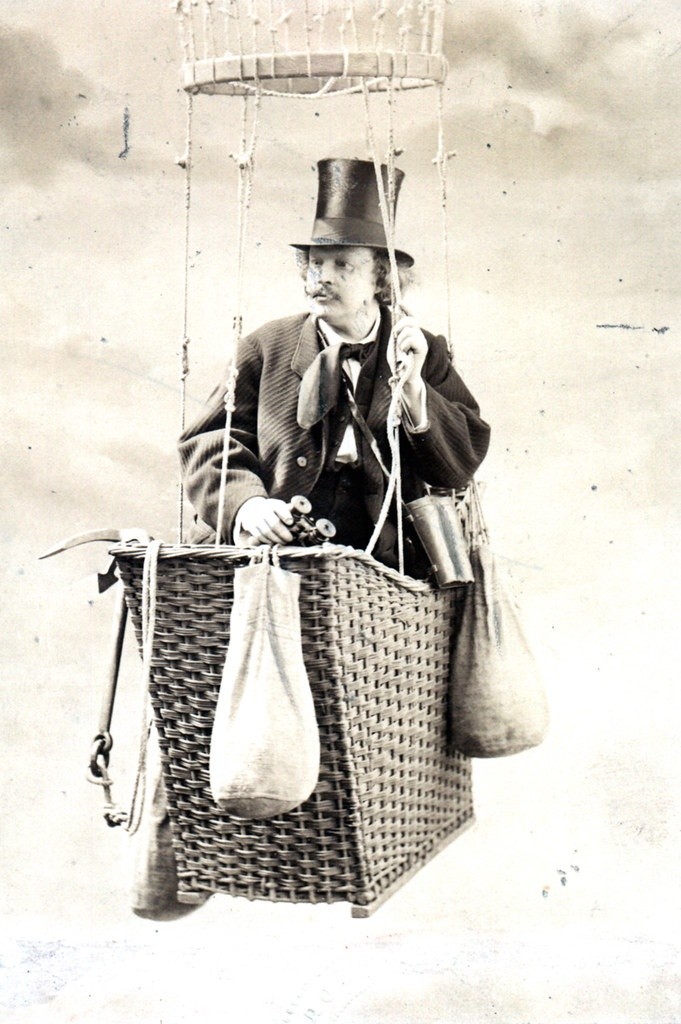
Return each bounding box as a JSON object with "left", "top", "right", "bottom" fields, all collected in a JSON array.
[{"left": 405, "top": 492, "right": 477, "bottom": 584}]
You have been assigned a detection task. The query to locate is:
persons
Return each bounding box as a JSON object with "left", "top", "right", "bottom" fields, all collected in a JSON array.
[{"left": 178, "top": 158, "right": 491, "bottom": 579}]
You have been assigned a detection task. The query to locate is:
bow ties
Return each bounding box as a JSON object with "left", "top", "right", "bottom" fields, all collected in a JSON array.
[{"left": 339, "top": 341, "right": 376, "bottom": 366}]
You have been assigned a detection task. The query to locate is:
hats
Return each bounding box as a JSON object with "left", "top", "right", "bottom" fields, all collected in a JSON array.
[{"left": 289, "top": 159, "right": 414, "bottom": 269}]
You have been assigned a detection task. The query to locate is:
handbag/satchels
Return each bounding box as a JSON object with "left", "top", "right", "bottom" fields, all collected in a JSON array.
[
  {"left": 316, "top": 329, "right": 475, "bottom": 589},
  {"left": 133, "top": 713, "right": 211, "bottom": 922},
  {"left": 207, "top": 542, "right": 320, "bottom": 819},
  {"left": 448, "top": 483, "right": 550, "bottom": 757}
]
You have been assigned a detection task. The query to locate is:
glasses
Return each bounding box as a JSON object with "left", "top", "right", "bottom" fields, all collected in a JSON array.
[{"left": 299, "top": 258, "right": 374, "bottom": 275}]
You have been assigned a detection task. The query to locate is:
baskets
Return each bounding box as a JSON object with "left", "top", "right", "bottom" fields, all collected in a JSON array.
[{"left": 106, "top": 539, "right": 476, "bottom": 917}]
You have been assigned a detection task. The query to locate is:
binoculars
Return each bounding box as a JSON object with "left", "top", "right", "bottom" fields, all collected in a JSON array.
[{"left": 260, "top": 494, "right": 337, "bottom": 545}]
